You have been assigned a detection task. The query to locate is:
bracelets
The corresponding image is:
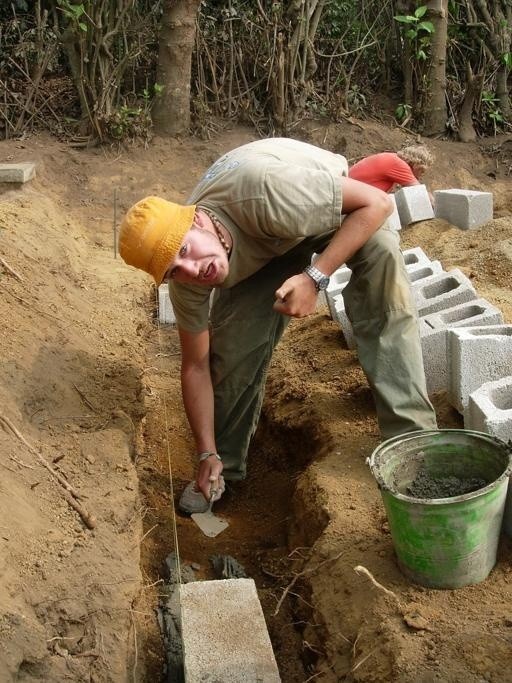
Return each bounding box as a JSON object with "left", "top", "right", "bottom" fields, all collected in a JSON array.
[{"left": 197, "top": 452, "right": 222, "bottom": 463}]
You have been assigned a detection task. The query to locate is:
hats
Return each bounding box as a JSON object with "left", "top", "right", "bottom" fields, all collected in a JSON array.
[{"left": 119, "top": 196, "right": 196, "bottom": 287}]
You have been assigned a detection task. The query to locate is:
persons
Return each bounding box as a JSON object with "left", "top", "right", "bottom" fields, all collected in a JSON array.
[
  {"left": 348, "top": 144, "right": 437, "bottom": 194},
  {"left": 114, "top": 135, "right": 439, "bottom": 502}
]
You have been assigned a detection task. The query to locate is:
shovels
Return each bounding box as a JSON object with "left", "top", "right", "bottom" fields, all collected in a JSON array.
[{"left": 191, "top": 480, "right": 229, "bottom": 538}]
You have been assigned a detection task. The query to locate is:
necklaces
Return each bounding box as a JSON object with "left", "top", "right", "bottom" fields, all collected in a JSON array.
[{"left": 199, "top": 206, "right": 232, "bottom": 253}]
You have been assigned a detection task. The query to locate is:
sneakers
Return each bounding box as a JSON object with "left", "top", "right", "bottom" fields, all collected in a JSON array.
[{"left": 178, "top": 480, "right": 209, "bottom": 513}]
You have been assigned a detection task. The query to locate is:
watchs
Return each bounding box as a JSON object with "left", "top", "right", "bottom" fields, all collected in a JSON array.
[{"left": 302, "top": 264, "right": 331, "bottom": 291}]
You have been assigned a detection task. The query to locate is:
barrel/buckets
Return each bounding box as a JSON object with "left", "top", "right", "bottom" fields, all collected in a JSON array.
[{"left": 365, "top": 428, "right": 512, "bottom": 592}]
